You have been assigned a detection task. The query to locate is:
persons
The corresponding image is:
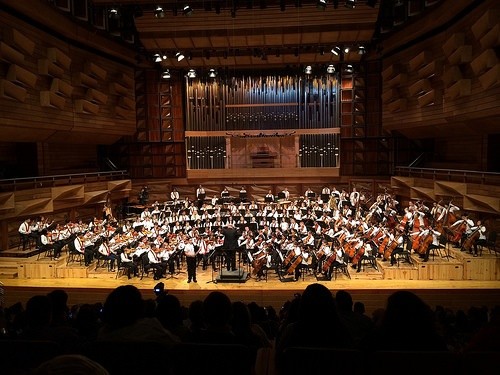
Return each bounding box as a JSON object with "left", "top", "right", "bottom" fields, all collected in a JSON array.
[
  {"left": 196, "top": 184, "right": 205, "bottom": 197},
  {"left": 222, "top": 224, "right": 242, "bottom": 271},
  {"left": 221, "top": 188, "right": 230, "bottom": 198},
  {"left": 0, "top": 283, "right": 500, "bottom": 375},
  {"left": 239, "top": 186, "right": 247, "bottom": 193},
  {"left": 262, "top": 185, "right": 486, "bottom": 279},
  {"left": 183, "top": 237, "right": 201, "bottom": 283},
  {"left": 18, "top": 195, "right": 272, "bottom": 281},
  {"left": 171, "top": 188, "right": 179, "bottom": 200}
]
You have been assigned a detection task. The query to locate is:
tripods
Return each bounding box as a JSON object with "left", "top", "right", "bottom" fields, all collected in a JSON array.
[
  {"left": 166, "top": 248, "right": 185, "bottom": 280},
  {"left": 207, "top": 250, "right": 217, "bottom": 284}
]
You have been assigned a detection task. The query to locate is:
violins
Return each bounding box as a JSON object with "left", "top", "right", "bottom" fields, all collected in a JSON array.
[{"left": 24, "top": 217, "right": 216, "bottom": 255}]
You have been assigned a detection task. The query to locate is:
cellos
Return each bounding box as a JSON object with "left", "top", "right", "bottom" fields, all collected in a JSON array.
[{"left": 251, "top": 196, "right": 486, "bottom": 279}]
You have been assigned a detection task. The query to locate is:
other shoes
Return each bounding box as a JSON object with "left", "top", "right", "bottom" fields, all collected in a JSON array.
[
  {"left": 187, "top": 280, "right": 191, "bottom": 283},
  {"left": 194, "top": 280, "right": 197, "bottom": 282}
]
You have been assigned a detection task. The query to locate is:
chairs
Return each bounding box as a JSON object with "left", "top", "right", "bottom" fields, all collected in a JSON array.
[{"left": 17, "top": 198, "right": 499, "bottom": 282}]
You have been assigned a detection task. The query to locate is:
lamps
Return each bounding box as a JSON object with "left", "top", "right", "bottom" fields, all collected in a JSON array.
[
  {"left": 317, "top": 0, "right": 358, "bottom": 13},
  {"left": 160, "top": 66, "right": 218, "bottom": 80},
  {"left": 150, "top": 52, "right": 185, "bottom": 63},
  {"left": 304, "top": 64, "right": 313, "bottom": 76},
  {"left": 327, "top": 63, "right": 353, "bottom": 74},
  {"left": 152, "top": 3, "right": 195, "bottom": 19},
  {"left": 105, "top": 5, "right": 121, "bottom": 21},
  {"left": 330, "top": 43, "right": 368, "bottom": 57}
]
